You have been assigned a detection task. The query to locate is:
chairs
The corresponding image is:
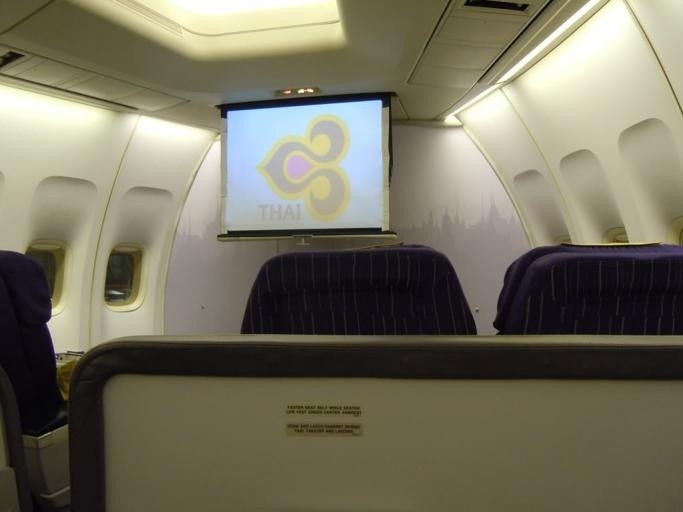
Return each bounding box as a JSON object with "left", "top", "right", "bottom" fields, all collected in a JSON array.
[
  {"left": 493, "top": 241, "right": 681, "bottom": 337},
  {"left": 240, "top": 243, "right": 479, "bottom": 339},
  {"left": 2, "top": 248, "right": 81, "bottom": 426}
]
